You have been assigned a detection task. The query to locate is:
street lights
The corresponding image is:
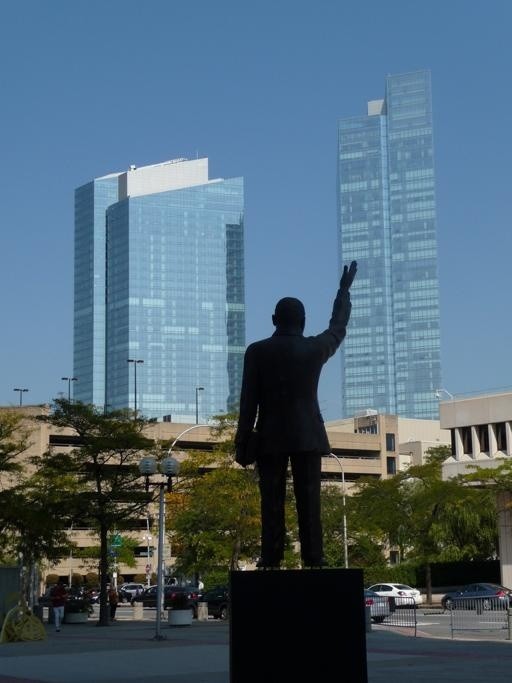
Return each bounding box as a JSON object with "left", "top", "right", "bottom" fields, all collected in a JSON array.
[
  {"left": 13, "top": 354, "right": 208, "bottom": 423},
  {"left": 161, "top": 420, "right": 235, "bottom": 619},
  {"left": 140, "top": 531, "right": 153, "bottom": 587},
  {"left": 132, "top": 450, "right": 182, "bottom": 641}
]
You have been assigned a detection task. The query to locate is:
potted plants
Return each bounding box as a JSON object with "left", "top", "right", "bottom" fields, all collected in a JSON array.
[
  {"left": 65, "top": 597, "right": 90, "bottom": 624},
  {"left": 167, "top": 594, "right": 195, "bottom": 626}
]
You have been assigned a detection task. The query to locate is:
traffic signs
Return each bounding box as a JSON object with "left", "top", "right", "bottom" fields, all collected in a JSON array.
[{"left": 109, "top": 533, "right": 122, "bottom": 547}]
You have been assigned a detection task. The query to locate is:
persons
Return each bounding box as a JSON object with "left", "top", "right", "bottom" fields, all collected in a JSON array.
[
  {"left": 231, "top": 257, "right": 360, "bottom": 570},
  {"left": 49, "top": 579, "right": 69, "bottom": 632},
  {"left": 109, "top": 584, "right": 120, "bottom": 621}
]
